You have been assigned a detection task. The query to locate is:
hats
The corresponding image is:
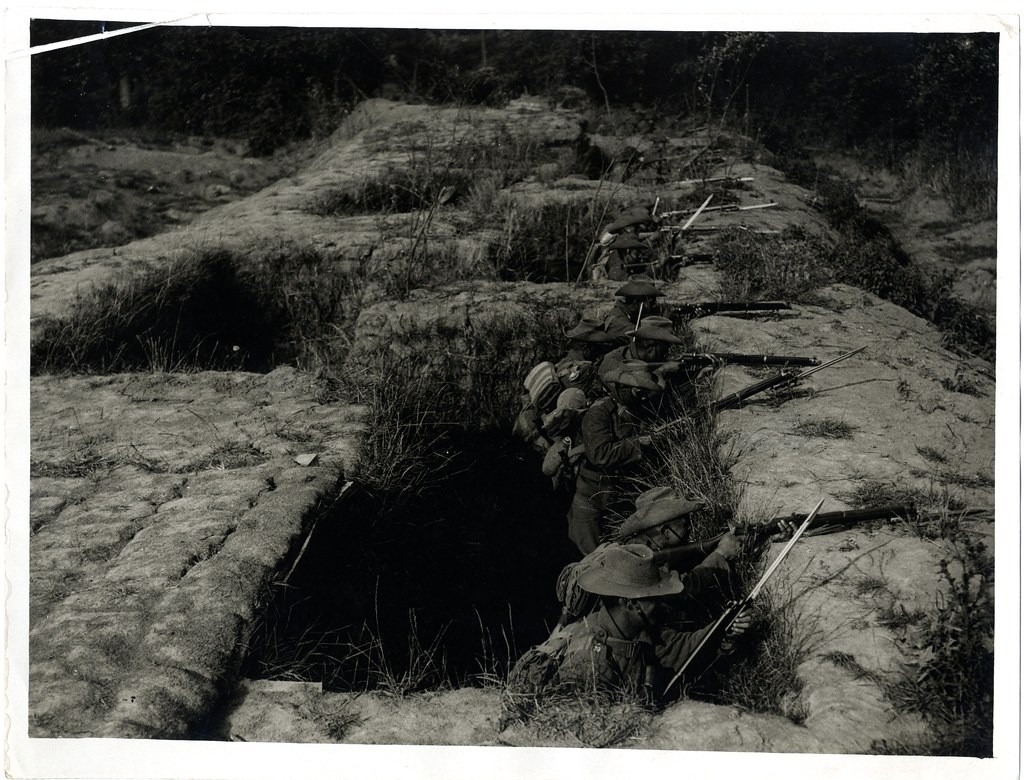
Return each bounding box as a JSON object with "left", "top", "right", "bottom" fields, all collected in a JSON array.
[
  {"left": 576, "top": 543, "right": 684, "bottom": 598},
  {"left": 603, "top": 359, "right": 666, "bottom": 391},
  {"left": 620, "top": 487, "right": 706, "bottom": 536},
  {"left": 625, "top": 316, "right": 684, "bottom": 345},
  {"left": 562, "top": 318, "right": 615, "bottom": 341},
  {"left": 614, "top": 274, "right": 668, "bottom": 297},
  {"left": 607, "top": 206, "right": 651, "bottom": 234},
  {"left": 608, "top": 234, "right": 648, "bottom": 250}
]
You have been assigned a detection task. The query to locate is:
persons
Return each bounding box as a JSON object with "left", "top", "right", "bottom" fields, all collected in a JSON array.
[
  {"left": 509, "top": 275, "right": 797, "bottom": 700},
  {"left": 610, "top": 109, "right": 671, "bottom": 185},
  {"left": 588, "top": 204, "right": 695, "bottom": 279}
]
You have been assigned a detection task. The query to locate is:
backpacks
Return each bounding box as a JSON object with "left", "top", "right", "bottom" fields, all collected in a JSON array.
[
  {"left": 591, "top": 227, "right": 615, "bottom": 283},
  {"left": 522, "top": 359, "right": 589, "bottom": 410},
  {"left": 555, "top": 538, "right": 622, "bottom": 617},
  {"left": 507, "top": 618, "right": 617, "bottom": 696}
]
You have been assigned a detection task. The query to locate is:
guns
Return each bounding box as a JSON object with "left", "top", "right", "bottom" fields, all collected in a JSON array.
[
  {"left": 658, "top": 497, "right": 826, "bottom": 702},
  {"left": 648, "top": 345, "right": 871, "bottom": 434},
  {"left": 620, "top": 250, "right": 719, "bottom": 274},
  {"left": 660, "top": 127, "right": 783, "bottom": 236},
  {"left": 654, "top": 497, "right": 987, "bottom": 568},
  {"left": 661, "top": 350, "right": 822, "bottom": 367},
  {"left": 656, "top": 299, "right": 792, "bottom": 319}
]
